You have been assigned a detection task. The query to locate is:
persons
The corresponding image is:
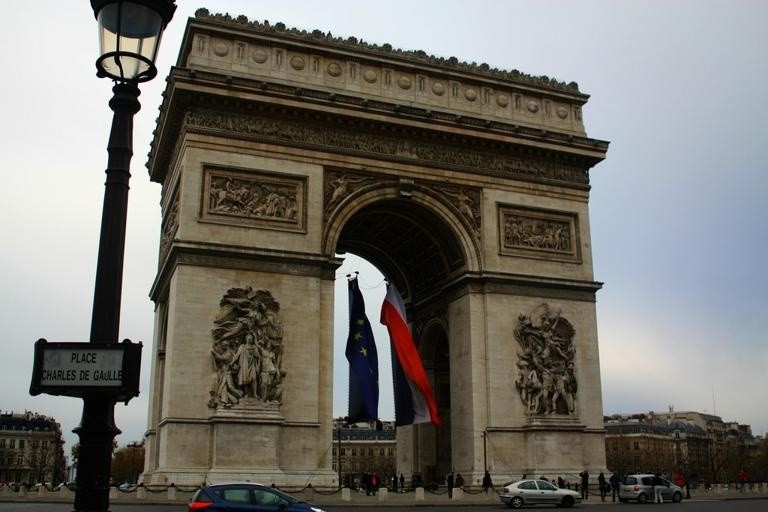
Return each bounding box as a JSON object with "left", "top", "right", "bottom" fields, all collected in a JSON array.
[
  {"left": 522, "top": 474, "right": 579, "bottom": 492},
  {"left": 577, "top": 470, "right": 589, "bottom": 499},
  {"left": 609, "top": 471, "right": 622, "bottom": 503},
  {"left": 210, "top": 294, "right": 285, "bottom": 411},
  {"left": 513, "top": 308, "right": 578, "bottom": 420},
  {"left": 483, "top": 471, "right": 493, "bottom": 491},
  {"left": 344, "top": 470, "right": 424, "bottom": 496},
  {"left": 597, "top": 472, "right": 607, "bottom": 503},
  {"left": 446, "top": 472, "right": 454, "bottom": 498},
  {"left": 654, "top": 473, "right": 663, "bottom": 504},
  {"left": 456, "top": 473, "right": 466, "bottom": 487}
]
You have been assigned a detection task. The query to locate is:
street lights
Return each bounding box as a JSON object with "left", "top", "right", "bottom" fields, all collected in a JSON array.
[{"left": 71, "top": 2, "right": 176, "bottom": 512}]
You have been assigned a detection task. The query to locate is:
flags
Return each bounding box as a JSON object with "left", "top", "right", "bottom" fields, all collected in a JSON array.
[
  {"left": 379, "top": 280, "right": 440, "bottom": 425},
  {"left": 344, "top": 275, "right": 378, "bottom": 422}
]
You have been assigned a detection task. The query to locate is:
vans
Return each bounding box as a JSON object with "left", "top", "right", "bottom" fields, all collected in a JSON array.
[{"left": 345, "top": 472, "right": 385, "bottom": 490}]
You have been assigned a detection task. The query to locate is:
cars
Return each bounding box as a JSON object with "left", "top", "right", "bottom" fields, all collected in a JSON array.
[
  {"left": 188, "top": 483, "right": 326, "bottom": 512},
  {"left": 500, "top": 480, "right": 583, "bottom": 508},
  {"left": 619, "top": 474, "right": 684, "bottom": 503}
]
